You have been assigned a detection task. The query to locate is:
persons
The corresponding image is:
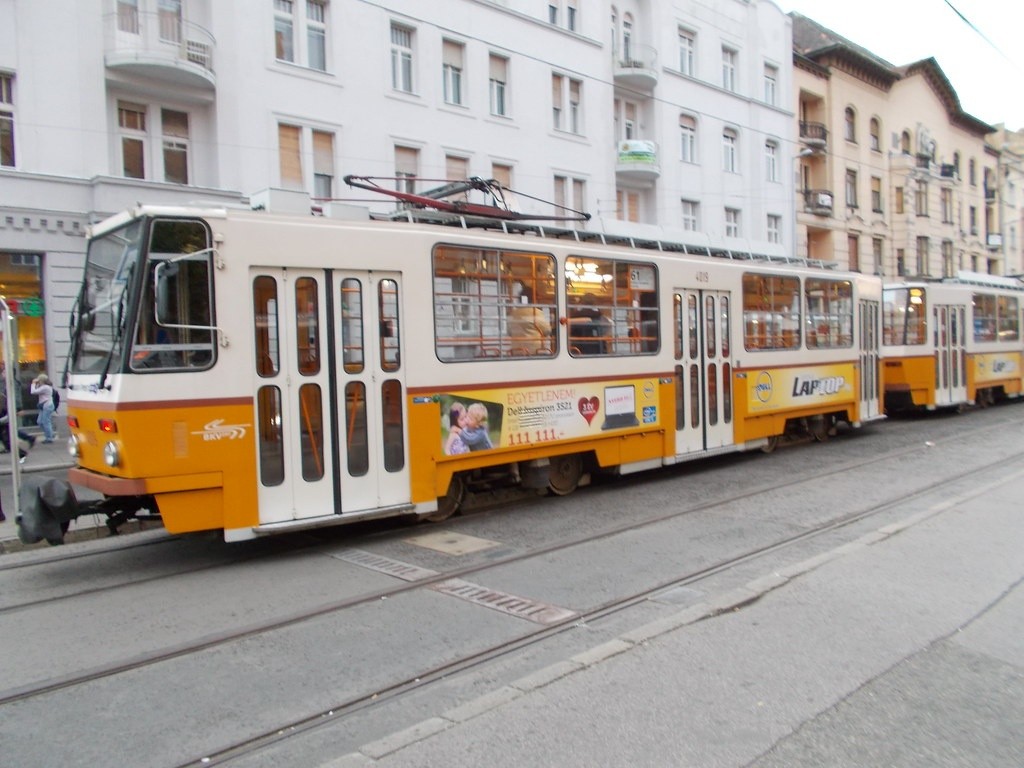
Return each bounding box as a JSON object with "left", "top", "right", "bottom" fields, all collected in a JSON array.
[
  {"left": 975, "top": 305, "right": 1009, "bottom": 336},
  {"left": 446, "top": 402, "right": 492, "bottom": 457},
  {"left": 508, "top": 287, "right": 606, "bottom": 358},
  {"left": 0, "top": 367, "right": 55, "bottom": 462}
]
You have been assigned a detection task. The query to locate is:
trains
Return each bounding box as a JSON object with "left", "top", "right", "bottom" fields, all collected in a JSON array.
[{"left": 9, "top": 170, "right": 1024, "bottom": 548}]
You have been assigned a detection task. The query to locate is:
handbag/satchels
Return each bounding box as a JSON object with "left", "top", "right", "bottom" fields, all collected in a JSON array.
[
  {"left": 36, "top": 400, "right": 48, "bottom": 410},
  {"left": 52, "top": 388, "right": 60, "bottom": 411}
]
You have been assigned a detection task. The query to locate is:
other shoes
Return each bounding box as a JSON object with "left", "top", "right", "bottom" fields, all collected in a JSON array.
[
  {"left": 0, "top": 449, "right": 10, "bottom": 453},
  {"left": 19, "top": 456, "right": 26, "bottom": 463},
  {"left": 42, "top": 440, "right": 53, "bottom": 443},
  {"left": 29, "top": 435, "right": 36, "bottom": 448}
]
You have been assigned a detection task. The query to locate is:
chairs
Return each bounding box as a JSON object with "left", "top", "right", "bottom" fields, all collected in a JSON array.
[{"left": 480, "top": 346, "right": 582, "bottom": 355}]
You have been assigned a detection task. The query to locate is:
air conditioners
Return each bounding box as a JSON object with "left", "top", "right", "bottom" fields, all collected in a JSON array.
[
  {"left": 986, "top": 232, "right": 1003, "bottom": 248},
  {"left": 816, "top": 194, "right": 832, "bottom": 208}
]
